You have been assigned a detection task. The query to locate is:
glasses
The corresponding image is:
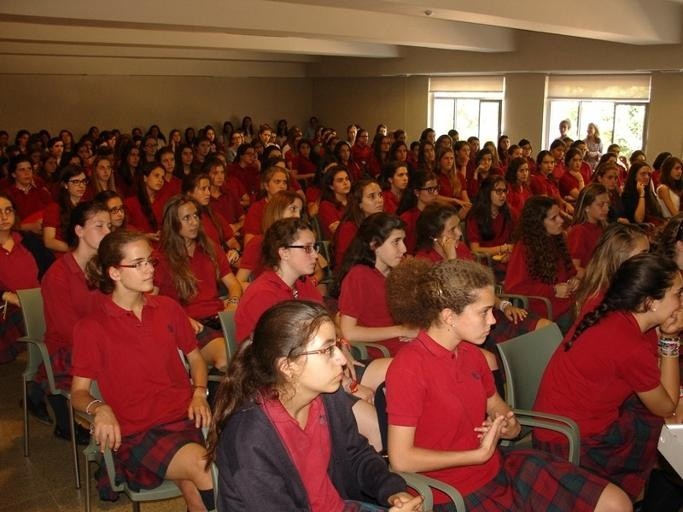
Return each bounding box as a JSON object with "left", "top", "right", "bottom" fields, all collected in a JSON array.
[
  {"left": 419, "top": 185, "right": 440, "bottom": 193},
  {"left": 109, "top": 206, "right": 126, "bottom": 214},
  {"left": 297, "top": 339, "right": 342, "bottom": 357},
  {"left": 287, "top": 245, "right": 320, "bottom": 255},
  {"left": 0, "top": 209, "right": 17, "bottom": 214},
  {"left": 114, "top": 257, "right": 159, "bottom": 269},
  {"left": 145, "top": 144, "right": 159, "bottom": 148},
  {"left": 69, "top": 178, "right": 89, "bottom": 186},
  {"left": 491, "top": 188, "right": 506, "bottom": 195}
]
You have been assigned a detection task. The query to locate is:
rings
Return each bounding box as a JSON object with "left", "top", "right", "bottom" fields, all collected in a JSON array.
[{"left": 434, "top": 238, "right": 439, "bottom": 242}]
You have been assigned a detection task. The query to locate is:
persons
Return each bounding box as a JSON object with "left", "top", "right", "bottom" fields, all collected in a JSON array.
[
  {"left": 67, "top": 229, "right": 216, "bottom": 511},
  {"left": 383, "top": 257, "right": 637, "bottom": 510},
  {"left": 3, "top": 111, "right": 682, "bottom": 463},
  {"left": 232, "top": 219, "right": 394, "bottom": 453},
  {"left": 532, "top": 253, "right": 681, "bottom": 510},
  {"left": 201, "top": 298, "right": 424, "bottom": 512}
]
